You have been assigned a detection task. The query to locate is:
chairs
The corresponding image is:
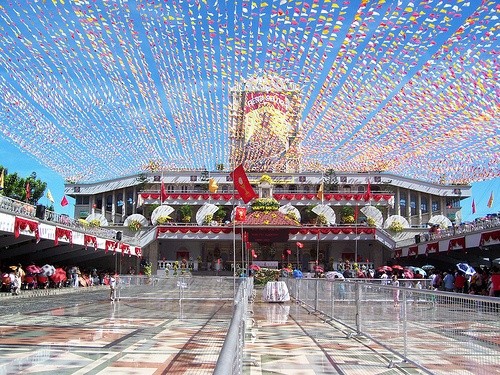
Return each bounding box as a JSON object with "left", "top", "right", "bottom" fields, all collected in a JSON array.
[{"left": 23, "top": 276, "right": 47, "bottom": 290}]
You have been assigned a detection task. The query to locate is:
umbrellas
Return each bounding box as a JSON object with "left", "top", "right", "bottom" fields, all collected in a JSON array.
[
  {"left": 0, "top": 264, "right": 68, "bottom": 284},
  {"left": 326, "top": 271, "right": 345, "bottom": 282},
  {"left": 456, "top": 263, "right": 476, "bottom": 277},
  {"left": 376, "top": 264, "right": 434, "bottom": 275}
]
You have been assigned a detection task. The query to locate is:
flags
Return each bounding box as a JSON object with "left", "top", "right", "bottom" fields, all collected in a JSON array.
[
  {"left": 61, "top": 196, "right": 69, "bottom": 206},
  {"left": 0, "top": 170, "right": 5, "bottom": 188},
  {"left": 317, "top": 183, "right": 323, "bottom": 199},
  {"left": 26, "top": 183, "right": 30, "bottom": 199},
  {"left": 46, "top": 191, "right": 54, "bottom": 203},
  {"left": 472, "top": 200, "right": 476, "bottom": 213},
  {"left": 160, "top": 182, "right": 169, "bottom": 203},
  {"left": 363, "top": 182, "right": 372, "bottom": 201},
  {"left": 487, "top": 194, "right": 494, "bottom": 208},
  {"left": 209, "top": 178, "right": 219, "bottom": 192}
]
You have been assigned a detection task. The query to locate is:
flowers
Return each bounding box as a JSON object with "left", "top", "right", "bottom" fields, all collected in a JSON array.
[
  {"left": 156, "top": 217, "right": 168, "bottom": 226},
  {"left": 390, "top": 218, "right": 403, "bottom": 232},
  {"left": 367, "top": 216, "right": 376, "bottom": 227},
  {"left": 344, "top": 214, "right": 355, "bottom": 224},
  {"left": 253, "top": 211, "right": 279, "bottom": 225},
  {"left": 284, "top": 212, "right": 301, "bottom": 226},
  {"left": 259, "top": 175, "right": 273, "bottom": 185},
  {"left": 252, "top": 197, "right": 279, "bottom": 210},
  {"left": 316, "top": 214, "right": 328, "bottom": 225},
  {"left": 89, "top": 219, "right": 101, "bottom": 229},
  {"left": 204, "top": 214, "right": 213, "bottom": 225},
  {"left": 127, "top": 222, "right": 141, "bottom": 232}
]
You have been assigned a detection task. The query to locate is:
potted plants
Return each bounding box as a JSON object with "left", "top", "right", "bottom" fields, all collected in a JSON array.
[
  {"left": 215, "top": 207, "right": 225, "bottom": 226},
  {"left": 178, "top": 204, "right": 193, "bottom": 223}
]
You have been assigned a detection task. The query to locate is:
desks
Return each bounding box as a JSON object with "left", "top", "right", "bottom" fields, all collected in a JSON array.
[{"left": 262, "top": 281, "right": 290, "bottom": 303}]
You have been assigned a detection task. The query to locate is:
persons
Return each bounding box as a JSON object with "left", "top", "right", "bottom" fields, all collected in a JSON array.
[
  {"left": 428, "top": 267, "right": 500, "bottom": 312},
  {"left": 391, "top": 275, "right": 400, "bottom": 307},
  {"left": 293, "top": 264, "right": 303, "bottom": 292},
  {"left": 64, "top": 266, "right": 115, "bottom": 287},
  {"left": 109, "top": 272, "right": 120, "bottom": 301},
  {"left": 335, "top": 266, "right": 427, "bottom": 300},
  {"left": 14, "top": 263, "right": 26, "bottom": 294}
]
